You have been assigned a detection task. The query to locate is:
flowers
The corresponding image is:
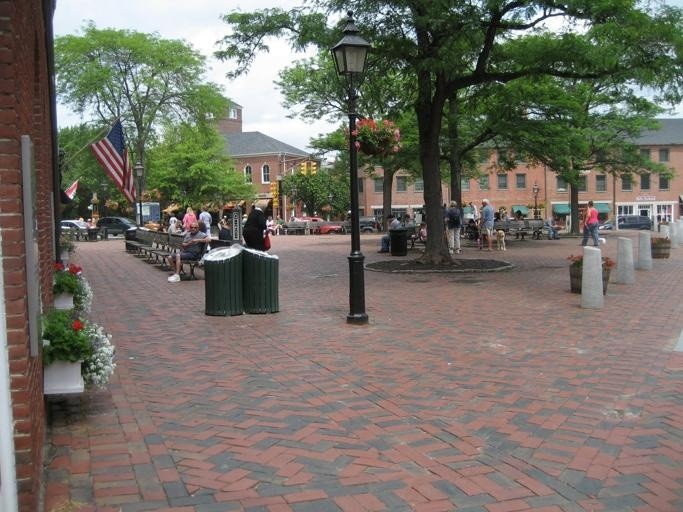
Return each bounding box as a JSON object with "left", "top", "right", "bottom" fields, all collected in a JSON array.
[
  {"left": 38, "top": 303, "right": 114, "bottom": 391},
  {"left": 650, "top": 236, "right": 668, "bottom": 242},
  {"left": 342, "top": 118, "right": 402, "bottom": 156},
  {"left": 566, "top": 252, "right": 617, "bottom": 269},
  {"left": 49, "top": 259, "right": 93, "bottom": 316}
]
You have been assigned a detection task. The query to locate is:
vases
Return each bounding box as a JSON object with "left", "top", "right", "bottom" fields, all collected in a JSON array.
[
  {"left": 569, "top": 264, "right": 611, "bottom": 295},
  {"left": 650, "top": 243, "right": 672, "bottom": 261}
]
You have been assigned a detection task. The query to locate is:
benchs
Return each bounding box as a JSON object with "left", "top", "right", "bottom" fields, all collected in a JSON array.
[
  {"left": 59, "top": 225, "right": 109, "bottom": 241},
  {"left": 403, "top": 217, "right": 545, "bottom": 251},
  {"left": 122, "top": 225, "right": 234, "bottom": 282}
]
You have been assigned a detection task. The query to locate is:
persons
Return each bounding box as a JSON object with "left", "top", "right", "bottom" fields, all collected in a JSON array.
[
  {"left": 77, "top": 216, "right": 100, "bottom": 228},
  {"left": 541, "top": 214, "right": 564, "bottom": 240},
  {"left": 377, "top": 197, "right": 526, "bottom": 254},
  {"left": 578, "top": 198, "right": 600, "bottom": 247},
  {"left": 658, "top": 218, "right": 669, "bottom": 225},
  {"left": 155, "top": 203, "right": 309, "bottom": 282}
]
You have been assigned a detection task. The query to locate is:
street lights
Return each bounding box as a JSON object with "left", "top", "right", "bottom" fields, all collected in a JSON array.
[
  {"left": 100, "top": 178, "right": 108, "bottom": 216},
  {"left": 329, "top": 11, "right": 370, "bottom": 326},
  {"left": 532, "top": 181, "right": 539, "bottom": 221},
  {"left": 133, "top": 160, "right": 144, "bottom": 227}
]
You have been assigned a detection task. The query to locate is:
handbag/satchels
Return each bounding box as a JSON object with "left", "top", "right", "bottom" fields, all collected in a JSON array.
[{"left": 263, "top": 234, "right": 272, "bottom": 251}]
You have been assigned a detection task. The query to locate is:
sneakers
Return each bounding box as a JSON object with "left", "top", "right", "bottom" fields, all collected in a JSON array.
[{"left": 167, "top": 273, "right": 181, "bottom": 282}]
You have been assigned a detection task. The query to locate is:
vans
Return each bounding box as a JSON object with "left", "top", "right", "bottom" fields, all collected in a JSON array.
[{"left": 598, "top": 215, "right": 652, "bottom": 231}]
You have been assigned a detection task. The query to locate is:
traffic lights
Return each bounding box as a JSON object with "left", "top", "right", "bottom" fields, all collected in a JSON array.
[
  {"left": 272, "top": 195, "right": 276, "bottom": 208},
  {"left": 310, "top": 161, "right": 316, "bottom": 175},
  {"left": 300, "top": 163, "right": 306, "bottom": 176},
  {"left": 270, "top": 182, "right": 276, "bottom": 194}
]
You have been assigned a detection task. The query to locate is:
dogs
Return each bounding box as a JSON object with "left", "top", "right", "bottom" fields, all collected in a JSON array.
[{"left": 496, "top": 230, "right": 507, "bottom": 252}]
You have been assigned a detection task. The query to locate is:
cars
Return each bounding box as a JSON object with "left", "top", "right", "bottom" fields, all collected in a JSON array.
[
  {"left": 59, "top": 219, "right": 95, "bottom": 233},
  {"left": 266, "top": 215, "right": 384, "bottom": 235}
]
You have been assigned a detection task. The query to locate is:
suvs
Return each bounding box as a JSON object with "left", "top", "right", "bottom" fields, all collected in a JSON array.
[{"left": 96, "top": 216, "right": 137, "bottom": 237}]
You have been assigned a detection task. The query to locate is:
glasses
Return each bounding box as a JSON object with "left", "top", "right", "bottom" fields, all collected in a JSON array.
[{"left": 190, "top": 226, "right": 199, "bottom": 230}]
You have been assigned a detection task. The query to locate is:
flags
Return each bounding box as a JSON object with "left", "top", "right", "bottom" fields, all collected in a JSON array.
[
  {"left": 62, "top": 176, "right": 80, "bottom": 200},
  {"left": 91, "top": 119, "right": 134, "bottom": 207}
]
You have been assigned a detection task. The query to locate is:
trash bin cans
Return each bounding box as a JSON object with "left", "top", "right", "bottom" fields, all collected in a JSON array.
[
  {"left": 203, "top": 245, "right": 280, "bottom": 316},
  {"left": 390, "top": 230, "right": 409, "bottom": 256},
  {"left": 88, "top": 229, "right": 97, "bottom": 241},
  {"left": 126, "top": 230, "right": 136, "bottom": 250}
]
